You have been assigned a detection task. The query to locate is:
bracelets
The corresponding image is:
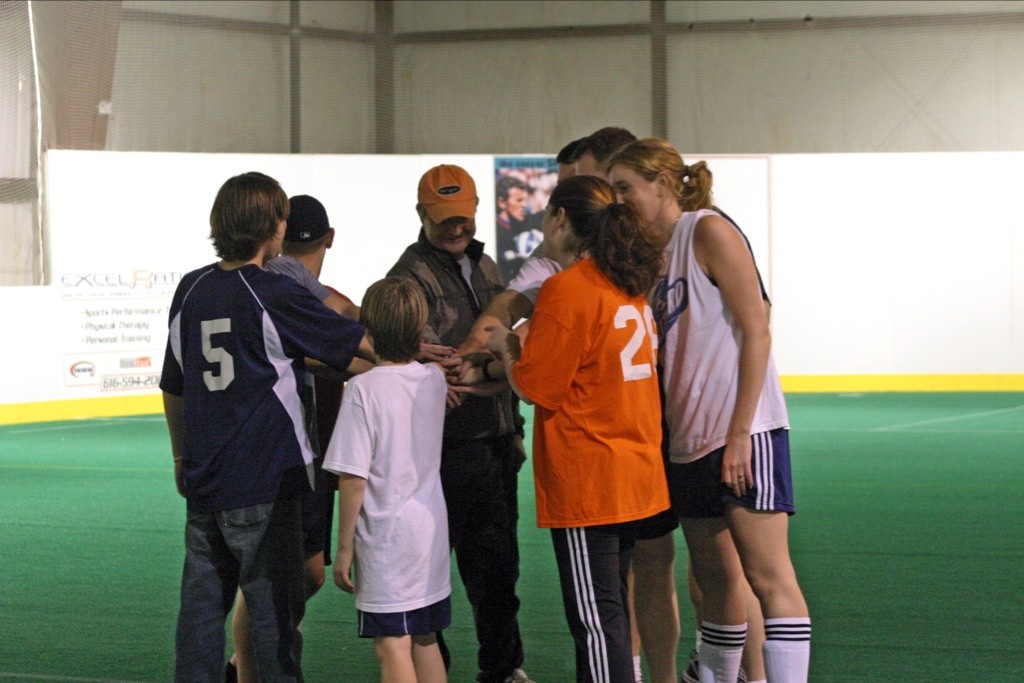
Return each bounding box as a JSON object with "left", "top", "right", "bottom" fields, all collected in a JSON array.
[{"left": 482, "top": 357, "right": 499, "bottom": 383}]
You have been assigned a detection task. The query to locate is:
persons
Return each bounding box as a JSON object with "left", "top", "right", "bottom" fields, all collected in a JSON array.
[
  {"left": 324, "top": 270, "right": 458, "bottom": 683},
  {"left": 157, "top": 126, "right": 814, "bottom": 683}
]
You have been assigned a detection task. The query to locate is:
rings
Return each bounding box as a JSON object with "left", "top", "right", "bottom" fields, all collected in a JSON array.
[{"left": 739, "top": 474, "right": 744, "bottom": 478}]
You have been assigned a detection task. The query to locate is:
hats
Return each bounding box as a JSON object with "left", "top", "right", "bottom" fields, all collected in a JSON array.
[
  {"left": 418, "top": 164, "right": 477, "bottom": 223},
  {"left": 284, "top": 195, "right": 330, "bottom": 243}
]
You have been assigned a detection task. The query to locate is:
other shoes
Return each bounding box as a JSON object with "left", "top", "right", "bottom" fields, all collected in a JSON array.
[
  {"left": 506, "top": 668, "right": 534, "bottom": 683},
  {"left": 681, "top": 651, "right": 747, "bottom": 683}
]
